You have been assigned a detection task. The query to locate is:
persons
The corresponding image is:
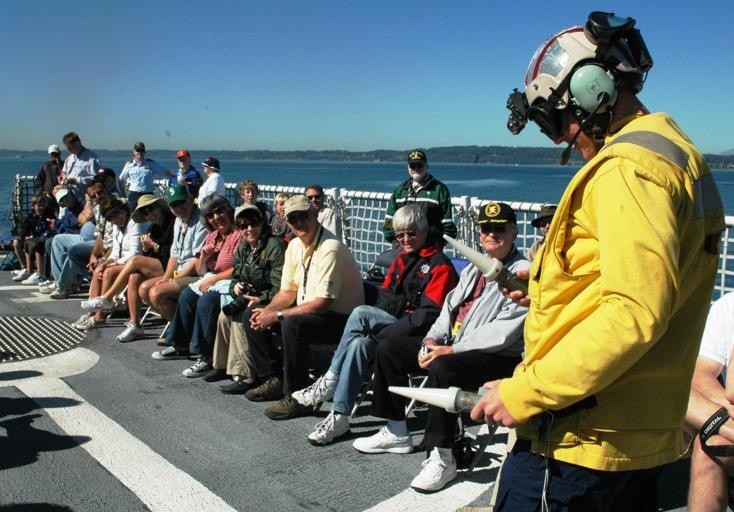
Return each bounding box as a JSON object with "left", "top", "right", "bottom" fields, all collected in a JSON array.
[
  {"left": 468, "top": 10, "right": 727, "bottom": 511},
  {"left": 686, "top": 293, "right": 733, "bottom": 512},
  {"left": 243, "top": 194, "right": 365, "bottom": 419},
  {"left": 291, "top": 203, "right": 460, "bottom": 446},
  {"left": 526, "top": 206, "right": 558, "bottom": 261},
  {"left": 151, "top": 195, "right": 244, "bottom": 378},
  {"left": 11, "top": 131, "right": 342, "bottom": 345},
  {"left": 382, "top": 150, "right": 457, "bottom": 249},
  {"left": 352, "top": 202, "right": 532, "bottom": 494},
  {"left": 202, "top": 203, "right": 287, "bottom": 394}
]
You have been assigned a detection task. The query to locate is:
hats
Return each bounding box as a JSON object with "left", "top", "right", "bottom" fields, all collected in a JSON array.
[
  {"left": 135, "top": 142, "right": 144, "bottom": 150},
  {"left": 283, "top": 194, "right": 311, "bottom": 218},
  {"left": 531, "top": 206, "right": 557, "bottom": 227},
  {"left": 177, "top": 150, "right": 190, "bottom": 158},
  {"left": 55, "top": 189, "right": 69, "bottom": 203},
  {"left": 94, "top": 167, "right": 115, "bottom": 179},
  {"left": 234, "top": 203, "right": 262, "bottom": 222},
  {"left": 201, "top": 158, "right": 220, "bottom": 171},
  {"left": 166, "top": 183, "right": 187, "bottom": 206},
  {"left": 131, "top": 194, "right": 163, "bottom": 223},
  {"left": 477, "top": 202, "right": 516, "bottom": 225},
  {"left": 48, "top": 145, "right": 59, "bottom": 154},
  {"left": 407, "top": 150, "right": 427, "bottom": 164},
  {"left": 309, "top": 195, "right": 320, "bottom": 200}
]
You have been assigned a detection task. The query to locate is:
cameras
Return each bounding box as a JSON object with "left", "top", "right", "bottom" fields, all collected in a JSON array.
[
  {"left": 61, "top": 175, "right": 67, "bottom": 185},
  {"left": 222, "top": 282, "right": 259, "bottom": 317}
]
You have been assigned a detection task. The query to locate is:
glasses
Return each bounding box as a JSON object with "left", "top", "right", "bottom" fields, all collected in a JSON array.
[
  {"left": 396, "top": 231, "right": 416, "bottom": 238},
  {"left": 528, "top": 105, "right": 565, "bottom": 140},
  {"left": 239, "top": 223, "right": 259, "bottom": 228},
  {"left": 288, "top": 213, "right": 309, "bottom": 224},
  {"left": 142, "top": 206, "right": 156, "bottom": 216}
]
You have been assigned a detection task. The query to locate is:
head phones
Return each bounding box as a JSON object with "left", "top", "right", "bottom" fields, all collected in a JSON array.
[{"left": 568, "top": 62, "right": 618, "bottom": 114}]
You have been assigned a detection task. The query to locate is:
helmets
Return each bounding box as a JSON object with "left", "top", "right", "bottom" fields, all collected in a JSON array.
[{"left": 524, "top": 26, "right": 643, "bottom": 110}]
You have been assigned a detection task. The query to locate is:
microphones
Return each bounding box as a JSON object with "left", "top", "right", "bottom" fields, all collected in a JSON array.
[{"left": 558, "top": 91, "right": 608, "bottom": 166}]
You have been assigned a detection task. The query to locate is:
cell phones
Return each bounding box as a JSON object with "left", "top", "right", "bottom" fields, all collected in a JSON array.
[{"left": 421, "top": 344, "right": 428, "bottom": 358}]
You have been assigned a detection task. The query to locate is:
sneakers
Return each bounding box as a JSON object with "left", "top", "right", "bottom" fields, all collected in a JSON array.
[
  {"left": 307, "top": 411, "right": 350, "bottom": 446},
  {"left": 411, "top": 446, "right": 457, "bottom": 494},
  {"left": 152, "top": 345, "right": 189, "bottom": 360},
  {"left": 183, "top": 358, "right": 283, "bottom": 401},
  {"left": 73, "top": 296, "right": 144, "bottom": 343},
  {"left": 291, "top": 375, "right": 338, "bottom": 408},
  {"left": 10, "top": 268, "right": 70, "bottom": 299},
  {"left": 353, "top": 427, "right": 415, "bottom": 455},
  {"left": 265, "top": 396, "right": 314, "bottom": 420}
]
